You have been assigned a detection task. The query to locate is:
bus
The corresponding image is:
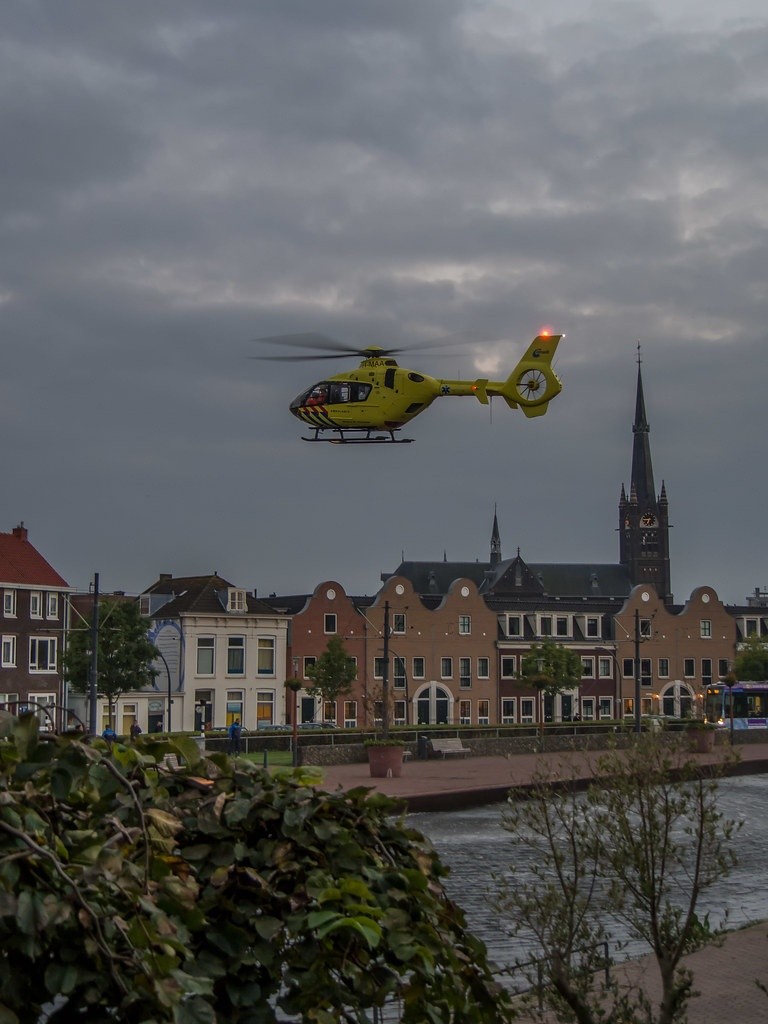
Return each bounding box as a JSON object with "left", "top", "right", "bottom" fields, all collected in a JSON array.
[{"left": 704, "top": 680, "right": 768, "bottom": 729}]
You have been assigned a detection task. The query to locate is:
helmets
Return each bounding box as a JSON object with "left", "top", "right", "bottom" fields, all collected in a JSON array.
[{"left": 319, "top": 385, "right": 326, "bottom": 391}]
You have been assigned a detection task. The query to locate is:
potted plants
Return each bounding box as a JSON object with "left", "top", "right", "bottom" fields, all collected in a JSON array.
[
  {"left": 685, "top": 723, "right": 719, "bottom": 753},
  {"left": 364, "top": 739, "right": 407, "bottom": 779}
]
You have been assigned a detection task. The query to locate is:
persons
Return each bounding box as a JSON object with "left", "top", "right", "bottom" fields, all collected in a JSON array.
[
  {"left": 75, "top": 725, "right": 82, "bottom": 730},
  {"left": 130, "top": 720, "right": 141, "bottom": 743},
  {"left": 573, "top": 713, "right": 581, "bottom": 721},
  {"left": 359, "top": 389, "right": 365, "bottom": 400},
  {"left": 102, "top": 725, "right": 117, "bottom": 744},
  {"left": 228, "top": 719, "right": 241, "bottom": 759},
  {"left": 306, "top": 389, "right": 328, "bottom": 405}
]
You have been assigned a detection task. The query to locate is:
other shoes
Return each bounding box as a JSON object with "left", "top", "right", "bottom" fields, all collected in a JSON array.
[{"left": 237, "top": 755, "right": 240, "bottom": 758}]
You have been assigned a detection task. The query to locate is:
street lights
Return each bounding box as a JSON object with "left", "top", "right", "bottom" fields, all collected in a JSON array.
[
  {"left": 378, "top": 648, "right": 409, "bottom": 725},
  {"left": 289, "top": 656, "right": 302, "bottom": 767}
]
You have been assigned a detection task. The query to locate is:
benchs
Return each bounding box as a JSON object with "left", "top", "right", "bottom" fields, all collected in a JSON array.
[
  {"left": 431, "top": 737, "right": 471, "bottom": 760},
  {"left": 403, "top": 751, "right": 412, "bottom": 763},
  {"left": 155, "top": 752, "right": 186, "bottom": 771}
]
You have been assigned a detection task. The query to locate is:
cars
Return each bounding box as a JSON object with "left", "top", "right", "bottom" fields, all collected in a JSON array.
[{"left": 213, "top": 722, "right": 341, "bottom": 730}]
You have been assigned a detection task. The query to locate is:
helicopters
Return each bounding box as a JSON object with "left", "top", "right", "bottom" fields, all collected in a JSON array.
[{"left": 248, "top": 332, "right": 563, "bottom": 444}]
